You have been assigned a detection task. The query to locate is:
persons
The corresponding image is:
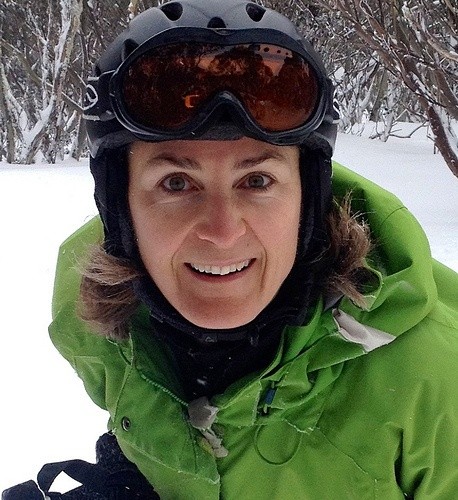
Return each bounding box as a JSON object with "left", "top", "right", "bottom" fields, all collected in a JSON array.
[{"left": 0, "top": 0, "right": 458, "bottom": 500}]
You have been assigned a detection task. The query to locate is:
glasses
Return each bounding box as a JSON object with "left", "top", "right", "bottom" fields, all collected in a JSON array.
[{"left": 108, "top": 26, "right": 333, "bottom": 146}]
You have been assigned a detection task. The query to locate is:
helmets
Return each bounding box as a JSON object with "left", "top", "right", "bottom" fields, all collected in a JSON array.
[{"left": 83, "top": 0, "right": 339, "bottom": 159}]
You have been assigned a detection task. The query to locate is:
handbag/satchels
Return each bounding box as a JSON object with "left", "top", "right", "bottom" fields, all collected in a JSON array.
[{"left": 1, "top": 459, "right": 148, "bottom": 500}]
